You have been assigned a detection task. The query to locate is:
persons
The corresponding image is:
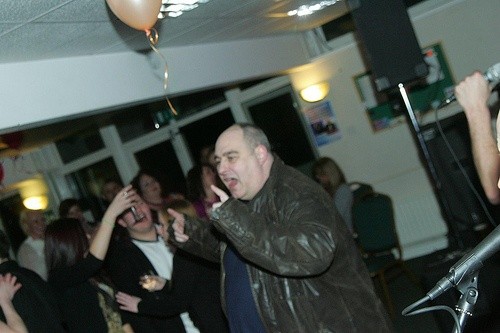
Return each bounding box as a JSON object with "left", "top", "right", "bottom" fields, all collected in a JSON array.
[
  {"left": 453, "top": 70, "right": 500, "bottom": 206},
  {"left": 168, "top": 121, "right": 394, "bottom": 333},
  {"left": 1, "top": 141, "right": 354, "bottom": 333}
]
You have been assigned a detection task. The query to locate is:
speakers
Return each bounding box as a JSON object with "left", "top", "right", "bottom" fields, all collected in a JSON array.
[{"left": 346, "top": 0, "right": 430, "bottom": 92}]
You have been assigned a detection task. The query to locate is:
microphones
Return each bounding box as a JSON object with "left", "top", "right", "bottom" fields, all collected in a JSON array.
[
  {"left": 440, "top": 63, "right": 500, "bottom": 109},
  {"left": 428, "top": 226, "right": 500, "bottom": 302}
]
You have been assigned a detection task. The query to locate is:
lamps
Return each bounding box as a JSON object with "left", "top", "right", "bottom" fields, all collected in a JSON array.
[
  {"left": 299, "top": 82, "right": 330, "bottom": 101},
  {"left": 19, "top": 178, "right": 49, "bottom": 209}
]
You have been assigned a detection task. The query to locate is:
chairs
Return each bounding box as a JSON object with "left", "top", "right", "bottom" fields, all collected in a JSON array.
[{"left": 349, "top": 182, "right": 403, "bottom": 293}]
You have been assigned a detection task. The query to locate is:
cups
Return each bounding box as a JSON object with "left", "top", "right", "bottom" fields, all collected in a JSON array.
[{"left": 124, "top": 188, "right": 147, "bottom": 222}]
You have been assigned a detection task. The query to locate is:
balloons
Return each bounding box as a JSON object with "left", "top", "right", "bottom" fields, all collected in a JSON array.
[{"left": 102, "top": 0, "right": 162, "bottom": 37}]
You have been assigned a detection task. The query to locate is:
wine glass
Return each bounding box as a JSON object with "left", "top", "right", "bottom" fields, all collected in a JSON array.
[{"left": 140, "top": 270, "right": 161, "bottom": 301}]
